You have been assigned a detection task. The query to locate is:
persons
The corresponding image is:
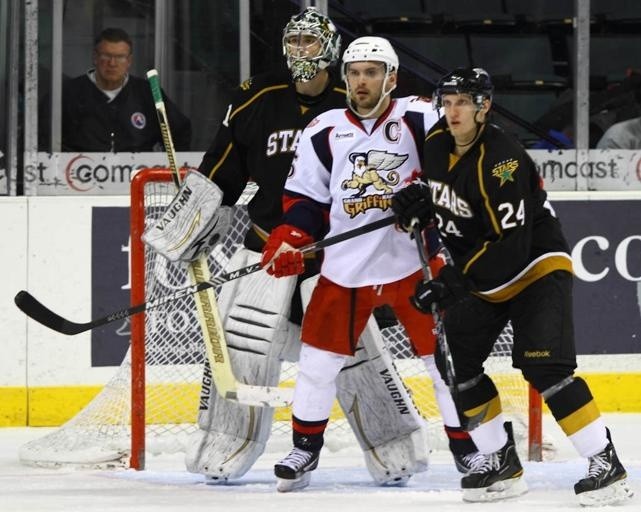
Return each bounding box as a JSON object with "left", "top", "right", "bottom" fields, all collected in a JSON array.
[
  {"left": 261, "top": 36, "right": 486, "bottom": 480},
  {"left": 595, "top": 117, "right": 641, "bottom": 149},
  {"left": 141, "top": 9, "right": 427, "bottom": 480},
  {"left": 532, "top": 116, "right": 575, "bottom": 149},
  {"left": 38, "top": 28, "right": 192, "bottom": 152},
  {"left": 392, "top": 67, "right": 627, "bottom": 495}
]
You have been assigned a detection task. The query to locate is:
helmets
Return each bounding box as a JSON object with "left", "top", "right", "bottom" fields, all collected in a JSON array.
[
  {"left": 281, "top": 8, "right": 341, "bottom": 83},
  {"left": 431, "top": 64, "right": 495, "bottom": 111},
  {"left": 340, "top": 36, "right": 399, "bottom": 81}
]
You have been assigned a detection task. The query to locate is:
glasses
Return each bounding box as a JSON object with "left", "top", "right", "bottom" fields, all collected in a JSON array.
[{"left": 92, "top": 48, "right": 131, "bottom": 62}]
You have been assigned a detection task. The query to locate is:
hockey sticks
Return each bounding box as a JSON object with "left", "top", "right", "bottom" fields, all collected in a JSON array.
[
  {"left": 148, "top": 70, "right": 296, "bottom": 407},
  {"left": 410, "top": 216, "right": 490, "bottom": 434},
  {"left": 14, "top": 213, "right": 396, "bottom": 334}
]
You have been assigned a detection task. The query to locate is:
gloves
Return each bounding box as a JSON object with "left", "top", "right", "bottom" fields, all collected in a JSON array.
[
  {"left": 409, "top": 264, "right": 469, "bottom": 314},
  {"left": 388, "top": 184, "right": 436, "bottom": 229},
  {"left": 260, "top": 224, "right": 315, "bottom": 276}
]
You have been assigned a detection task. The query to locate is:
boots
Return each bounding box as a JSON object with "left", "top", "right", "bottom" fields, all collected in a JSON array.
[
  {"left": 572, "top": 426, "right": 629, "bottom": 495},
  {"left": 273, "top": 410, "right": 331, "bottom": 479},
  {"left": 442, "top": 421, "right": 525, "bottom": 489}
]
